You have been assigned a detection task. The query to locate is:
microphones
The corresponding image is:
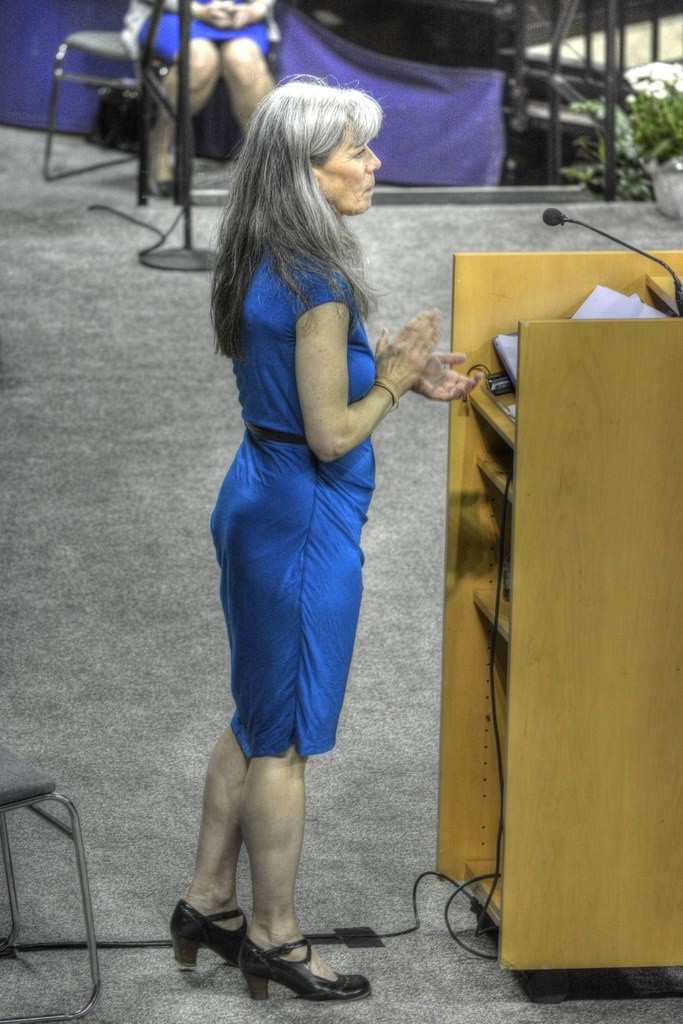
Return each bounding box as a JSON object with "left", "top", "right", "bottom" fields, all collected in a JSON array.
[{"left": 543, "top": 208, "right": 683, "bottom": 318}]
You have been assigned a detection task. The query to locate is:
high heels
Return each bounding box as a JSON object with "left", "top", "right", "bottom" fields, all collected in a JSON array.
[
  {"left": 238, "top": 935, "right": 372, "bottom": 1002},
  {"left": 170, "top": 900, "right": 249, "bottom": 968}
]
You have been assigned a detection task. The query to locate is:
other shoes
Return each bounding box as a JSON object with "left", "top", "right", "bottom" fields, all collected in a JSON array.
[{"left": 156, "top": 177, "right": 174, "bottom": 197}]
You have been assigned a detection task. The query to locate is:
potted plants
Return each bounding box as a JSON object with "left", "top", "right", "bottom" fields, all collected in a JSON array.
[{"left": 624, "top": 70, "right": 683, "bottom": 219}]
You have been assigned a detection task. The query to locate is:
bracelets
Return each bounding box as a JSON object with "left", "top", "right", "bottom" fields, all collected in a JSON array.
[{"left": 373, "top": 379, "right": 400, "bottom": 408}]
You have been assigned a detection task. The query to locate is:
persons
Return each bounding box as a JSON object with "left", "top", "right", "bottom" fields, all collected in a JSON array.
[
  {"left": 137, "top": 0, "right": 282, "bottom": 192},
  {"left": 172, "top": 75, "right": 481, "bottom": 998}
]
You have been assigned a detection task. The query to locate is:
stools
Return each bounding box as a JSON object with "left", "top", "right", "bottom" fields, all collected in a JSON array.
[
  {"left": 0, "top": 745, "right": 101, "bottom": 1024},
  {"left": 44, "top": 31, "right": 282, "bottom": 198}
]
